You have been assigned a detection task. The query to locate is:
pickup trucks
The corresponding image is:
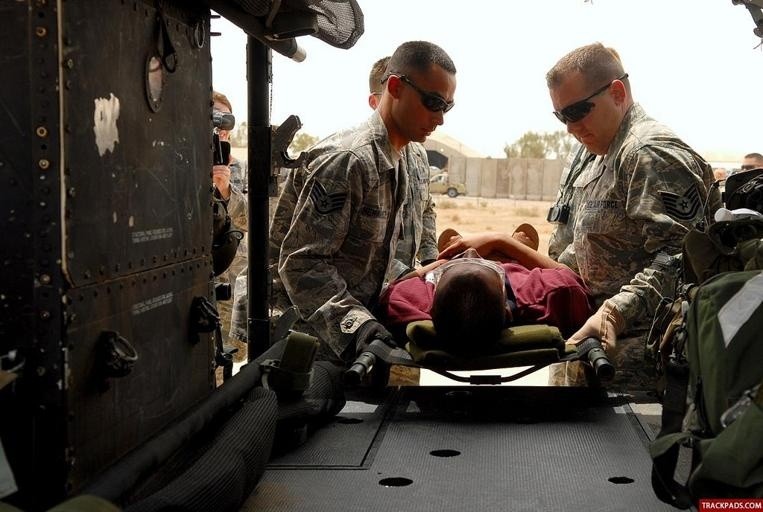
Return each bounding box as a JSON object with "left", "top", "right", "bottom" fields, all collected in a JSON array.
[{"left": 427, "top": 170, "right": 468, "bottom": 198}]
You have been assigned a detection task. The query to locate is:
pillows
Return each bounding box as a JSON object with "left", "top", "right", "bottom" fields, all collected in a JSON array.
[{"left": 406, "top": 320, "right": 565, "bottom": 364}]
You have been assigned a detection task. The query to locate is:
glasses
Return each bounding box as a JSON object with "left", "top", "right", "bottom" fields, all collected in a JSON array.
[
  {"left": 378, "top": 72, "right": 455, "bottom": 116},
  {"left": 740, "top": 163, "right": 759, "bottom": 172},
  {"left": 549, "top": 72, "right": 631, "bottom": 125}
]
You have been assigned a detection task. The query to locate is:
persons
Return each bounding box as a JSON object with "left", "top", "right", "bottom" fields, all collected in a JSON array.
[
  {"left": 381, "top": 223, "right": 596, "bottom": 369},
  {"left": 545, "top": 41, "right": 725, "bottom": 397},
  {"left": 740, "top": 151, "right": 762, "bottom": 175},
  {"left": 366, "top": 54, "right": 440, "bottom": 268},
  {"left": 213, "top": 91, "right": 248, "bottom": 388},
  {"left": 227, "top": 41, "right": 457, "bottom": 370}
]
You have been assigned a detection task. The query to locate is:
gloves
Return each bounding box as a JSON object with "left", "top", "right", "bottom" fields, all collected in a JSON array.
[
  {"left": 565, "top": 297, "right": 625, "bottom": 363},
  {"left": 349, "top": 322, "right": 399, "bottom": 376}
]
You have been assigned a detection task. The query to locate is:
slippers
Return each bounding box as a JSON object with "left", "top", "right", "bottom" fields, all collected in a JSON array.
[
  {"left": 510, "top": 222, "right": 544, "bottom": 253},
  {"left": 434, "top": 227, "right": 465, "bottom": 255}
]
